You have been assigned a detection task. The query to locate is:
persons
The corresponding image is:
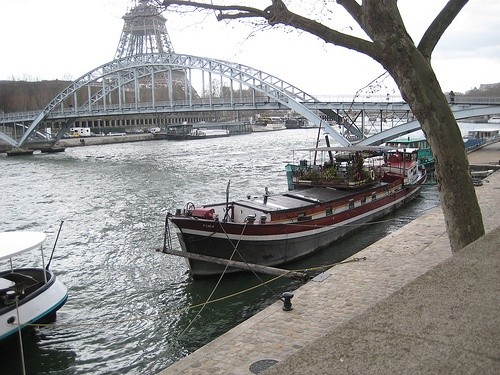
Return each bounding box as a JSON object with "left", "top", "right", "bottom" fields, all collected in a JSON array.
[{"left": 449, "top": 91, "right": 455, "bottom": 101}]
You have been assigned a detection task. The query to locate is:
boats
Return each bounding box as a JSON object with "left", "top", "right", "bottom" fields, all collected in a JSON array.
[
  {"left": 158, "top": 135, "right": 429, "bottom": 280},
  {"left": 0, "top": 221, "right": 69, "bottom": 340}
]
